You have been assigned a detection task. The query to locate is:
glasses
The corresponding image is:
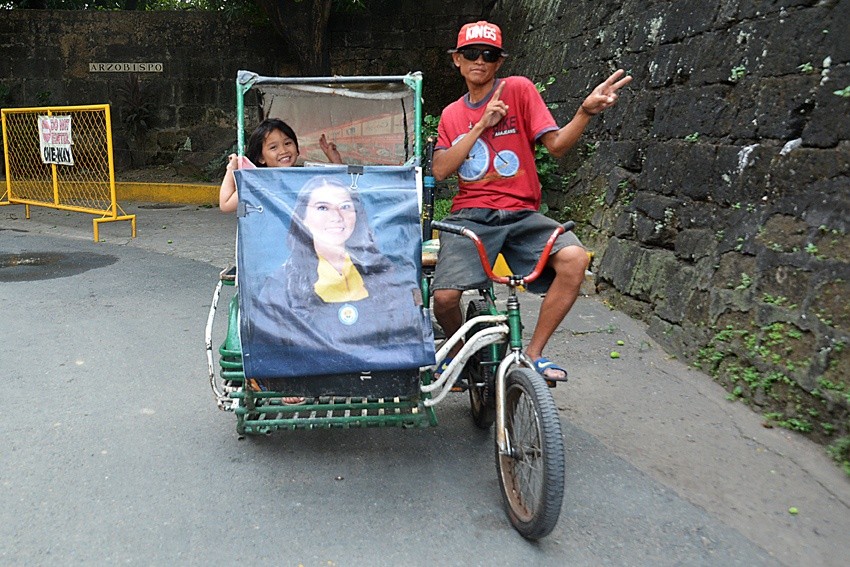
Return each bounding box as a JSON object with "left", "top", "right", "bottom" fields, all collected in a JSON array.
[{"left": 457, "top": 46, "right": 502, "bottom": 63}]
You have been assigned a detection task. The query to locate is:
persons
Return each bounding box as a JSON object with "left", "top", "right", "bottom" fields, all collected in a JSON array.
[
  {"left": 218, "top": 118, "right": 342, "bottom": 212},
  {"left": 433, "top": 21, "right": 631, "bottom": 393},
  {"left": 250, "top": 177, "right": 430, "bottom": 346}
]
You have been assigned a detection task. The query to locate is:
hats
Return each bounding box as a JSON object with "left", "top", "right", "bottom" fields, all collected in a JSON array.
[{"left": 455, "top": 20, "right": 505, "bottom": 53}]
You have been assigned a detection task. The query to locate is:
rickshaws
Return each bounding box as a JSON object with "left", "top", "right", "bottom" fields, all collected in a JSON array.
[{"left": 202, "top": 67, "right": 578, "bottom": 543}]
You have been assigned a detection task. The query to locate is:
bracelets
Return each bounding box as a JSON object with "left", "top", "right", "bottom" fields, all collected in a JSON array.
[{"left": 580, "top": 102, "right": 597, "bottom": 116}]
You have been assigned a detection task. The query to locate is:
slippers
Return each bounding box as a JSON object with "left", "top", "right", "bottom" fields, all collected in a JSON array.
[
  {"left": 520, "top": 357, "right": 568, "bottom": 382},
  {"left": 431, "top": 356, "right": 453, "bottom": 383}
]
